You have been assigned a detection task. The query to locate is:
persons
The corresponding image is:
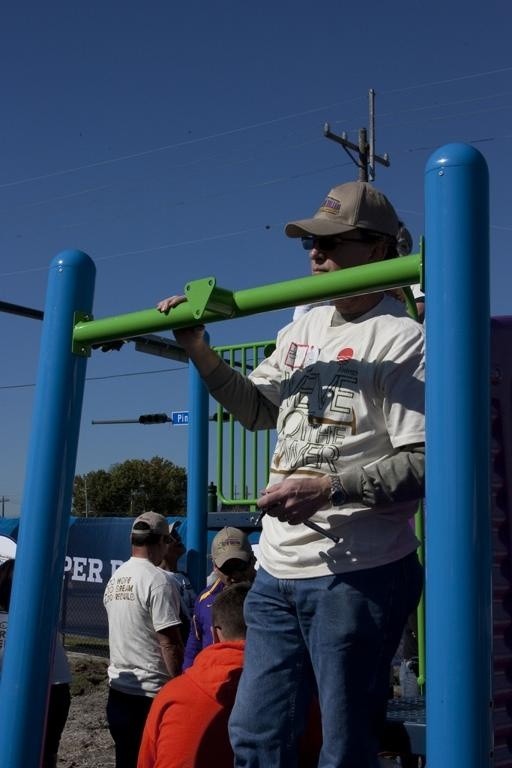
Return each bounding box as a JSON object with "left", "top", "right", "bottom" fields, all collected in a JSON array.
[
  {"left": 2, "top": 556, "right": 72, "bottom": 766},
  {"left": 103, "top": 511, "right": 184, "bottom": 765},
  {"left": 138, "top": 580, "right": 322, "bottom": 767},
  {"left": 182, "top": 525, "right": 256, "bottom": 669},
  {"left": 391, "top": 610, "right": 424, "bottom": 766},
  {"left": 157, "top": 520, "right": 198, "bottom": 625},
  {"left": 154, "top": 182, "right": 425, "bottom": 766}
]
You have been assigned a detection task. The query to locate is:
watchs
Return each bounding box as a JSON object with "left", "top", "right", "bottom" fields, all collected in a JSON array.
[{"left": 327, "top": 474, "right": 347, "bottom": 510}]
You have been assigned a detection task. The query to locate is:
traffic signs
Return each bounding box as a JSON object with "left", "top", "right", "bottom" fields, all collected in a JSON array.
[{"left": 172, "top": 411, "right": 188, "bottom": 425}]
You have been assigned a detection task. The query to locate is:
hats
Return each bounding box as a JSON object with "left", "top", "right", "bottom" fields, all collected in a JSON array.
[
  {"left": 211, "top": 527, "right": 251, "bottom": 569},
  {"left": 0, "top": 535, "right": 17, "bottom": 566},
  {"left": 169, "top": 520, "right": 182, "bottom": 534},
  {"left": 284, "top": 180, "right": 399, "bottom": 237},
  {"left": 132, "top": 512, "right": 177, "bottom": 543}
]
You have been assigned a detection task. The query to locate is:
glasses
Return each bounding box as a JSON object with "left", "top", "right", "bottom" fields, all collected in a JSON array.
[{"left": 302, "top": 233, "right": 386, "bottom": 251}]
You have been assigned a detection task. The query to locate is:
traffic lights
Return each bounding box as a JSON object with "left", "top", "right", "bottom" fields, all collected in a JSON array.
[{"left": 139, "top": 413, "right": 168, "bottom": 424}]
